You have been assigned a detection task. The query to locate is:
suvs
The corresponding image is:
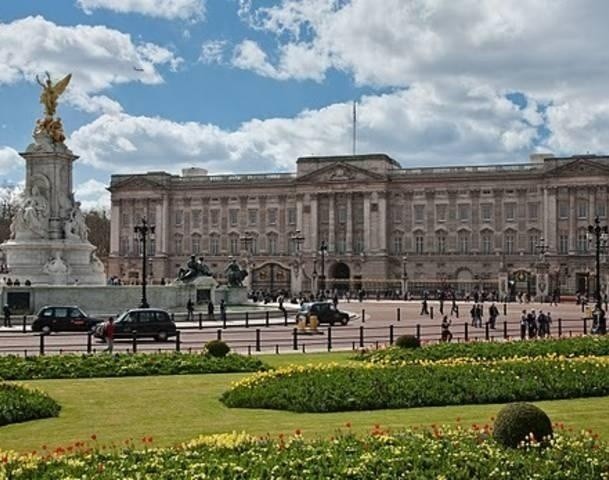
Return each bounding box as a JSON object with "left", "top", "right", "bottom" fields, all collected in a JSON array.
[
  {"left": 296, "top": 302, "right": 349, "bottom": 325},
  {"left": 95, "top": 308, "right": 177, "bottom": 343},
  {"left": 33, "top": 305, "right": 105, "bottom": 337}
]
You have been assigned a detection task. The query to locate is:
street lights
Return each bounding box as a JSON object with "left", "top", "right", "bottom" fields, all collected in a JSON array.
[
  {"left": 134, "top": 217, "right": 157, "bottom": 308},
  {"left": 292, "top": 228, "right": 305, "bottom": 251},
  {"left": 537, "top": 237, "right": 548, "bottom": 302},
  {"left": 318, "top": 240, "right": 327, "bottom": 291},
  {"left": 240, "top": 230, "right": 254, "bottom": 250},
  {"left": 148, "top": 256, "right": 154, "bottom": 279},
  {"left": 402, "top": 254, "right": 408, "bottom": 295},
  {"left": 586, "top": 215, "right": 609, "bottom": 337}
]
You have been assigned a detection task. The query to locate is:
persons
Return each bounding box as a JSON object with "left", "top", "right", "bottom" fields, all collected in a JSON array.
[
  {"left": 104, "top": 317, "right": 115, "bottom": 352},
  {"left": 186, "top": 299, "right": 196, "bottom": 322},
  {"left": 220, "top": 300, "right": 226, "bottom": 320},
  {"left": 197, "top": 257, "right": 210, "bottom": 271},
  {"left": 207, "top": 301, "right": 215, "bottom": 319},
  {"left": 105, "top": 274, "right": 167, "bottom": 286},
  {"left": 248, "top": 288, "right": 414, "bottom": 317},
  {"left": 224, "top": 259, "right": 242, "bottom": 287},
  {"left": 0, "top": 277, "right": 31, "bottom": 297},
  {"left": 419, "top": 288, "right": 608, "bottom": 342},
  {"left": 3, "top": 303, "right": 12, "bottom": 328},
  {"left": 42, "top": 80, "right": 56, "bottom": 113},
  {"left": 175, "top": 254, "right": 195, "bottom": 281}
]
